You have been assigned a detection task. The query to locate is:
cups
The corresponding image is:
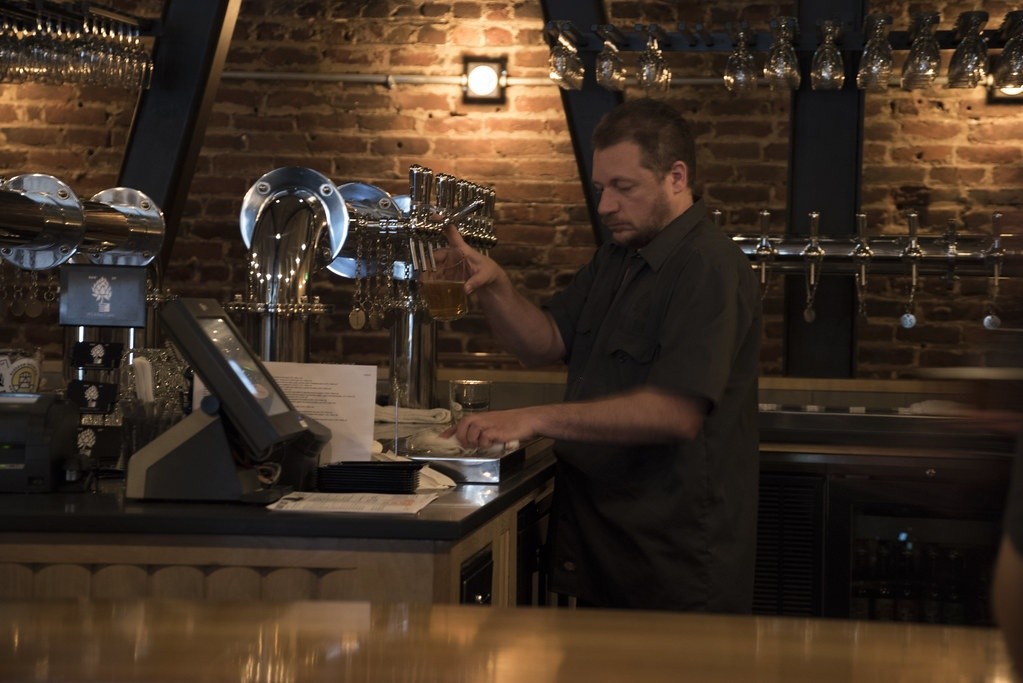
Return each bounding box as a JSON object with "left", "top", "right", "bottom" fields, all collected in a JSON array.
[
  {"left": 450, "top": 378, "right": 492, "bottom": 426},
  {"left": 0, "top": 341, "right": 45, "bottom": 396},
  {"left": 422, "top": 245, "right": 470, "bottom": 322},
  {"left": 116, "top": 343, "right": 183, "bottom": 415}
]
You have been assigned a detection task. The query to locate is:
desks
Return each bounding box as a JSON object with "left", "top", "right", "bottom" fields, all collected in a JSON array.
[
  {"left": 755, "top": 431, "right": 1023, "bottom": 482},
  {"left": 2, "top": 409, "right": 563, "bottom": 609},
  {"left": 0, "top": 595, "right": 1020, "bottom": 683}
]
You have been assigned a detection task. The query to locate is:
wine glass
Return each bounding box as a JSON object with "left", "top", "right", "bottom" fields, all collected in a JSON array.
[
  {"left": 709, "top": 14, "right": 1022, "bottom": 94},
  {"left": 0, "top": 6, "right": 155, "bottom": 98},
  {"left": 547, "top": 19, "right": 673, "bottom": 102}
]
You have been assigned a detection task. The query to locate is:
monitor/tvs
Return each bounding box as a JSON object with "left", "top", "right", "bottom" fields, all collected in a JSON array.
[{"left": 158, "top": 296, "right": 309, "bottom": 453}]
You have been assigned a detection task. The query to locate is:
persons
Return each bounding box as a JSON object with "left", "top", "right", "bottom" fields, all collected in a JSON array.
[{"left": 419, "top": 99, "right": 763, "bottom": 615}]
